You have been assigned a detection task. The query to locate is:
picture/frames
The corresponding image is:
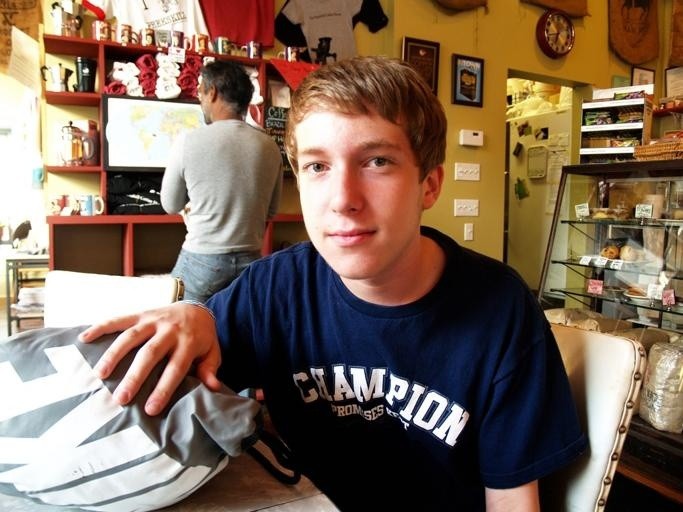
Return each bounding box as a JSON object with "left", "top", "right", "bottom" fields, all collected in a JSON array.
[
  {"left": 631, "top": 66, "right": 656, "bottom": 86},
  {"left": 452, "top": 53, "right": 484, "bottom": 108},
  {"left": 665, "top": 66, "right": 683, "bottom": 97},
  {"left": 402, "top": 36, "right": 440, "bottom": 97}
]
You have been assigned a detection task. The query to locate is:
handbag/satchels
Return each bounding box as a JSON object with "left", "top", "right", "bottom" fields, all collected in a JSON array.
[{"left": 0, "top": 326, "right": 299, "bottom": 512}]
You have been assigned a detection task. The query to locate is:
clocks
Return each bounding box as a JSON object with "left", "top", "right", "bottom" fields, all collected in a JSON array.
[{"left": 536, "top": 10, "right": 575, "bottom": 59}]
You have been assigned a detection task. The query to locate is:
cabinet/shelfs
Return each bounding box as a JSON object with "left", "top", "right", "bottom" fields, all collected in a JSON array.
[
  {"left": 537, "top": 158, "right": 683, "bottom": 333},
  {"left": 43, "top": 34, "right": 323, "bottom": 277}
]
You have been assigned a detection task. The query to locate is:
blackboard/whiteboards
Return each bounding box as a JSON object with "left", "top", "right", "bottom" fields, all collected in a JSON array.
[{"left": 264, "top": 105, "right": 296, "bottom": 178}]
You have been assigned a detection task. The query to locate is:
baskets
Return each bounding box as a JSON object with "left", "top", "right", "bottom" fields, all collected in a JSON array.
[{"left": 633, "top": 142, "right": 683, "bottom": 161}]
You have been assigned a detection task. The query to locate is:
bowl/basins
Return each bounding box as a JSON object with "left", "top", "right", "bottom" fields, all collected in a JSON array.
[{"left": 622, "top": 293, "right": 654, "bottom": 305}]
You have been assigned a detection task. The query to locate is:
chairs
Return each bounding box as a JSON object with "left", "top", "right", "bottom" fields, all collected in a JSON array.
[{"left": 542, "top": 324, "right": 647, "bottom": 512}]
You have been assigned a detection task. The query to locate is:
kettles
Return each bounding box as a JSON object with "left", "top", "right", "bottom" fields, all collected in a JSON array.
[
  {"left": 61, "top": 121, "right": 94, "bottom": 166},
  {"left": 41, "top": 64, "right": 73, "bottom": 92}
]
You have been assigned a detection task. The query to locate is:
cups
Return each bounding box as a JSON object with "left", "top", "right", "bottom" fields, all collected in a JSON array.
[
  {"left": 120, "top": 25, "right": 139, "bottom": 43},
  {"left": 277, "top": 46, "right": 298, "bottom": 62},
  {"left": 146, "top": 29, "right": 211, "bottom": 52},
  {"left": 643, "top": 194, "right": 668, "bottom": 219},
  {"left": 79, "top": 193, "right": 104, "bottom": 215},
  {"left": 74, "top": 57, "right": 95, "bottom": 94},
  {"left": 216, "top": 36, "right": 237, "bottom": 54},
  {"left": 93, "top": 20, "right": 113, "bottom": 41},
  {"left": 637, "top": 307, "right": 646, "bottom": 320},
  {"left": 239, "top": 41, "right": 263, "bottom": 59}
]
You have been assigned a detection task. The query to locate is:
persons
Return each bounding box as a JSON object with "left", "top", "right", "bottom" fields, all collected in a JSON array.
[
  {"left": 77, "top": 56, "right": 591, "bottom": 511},
  {"left": 158, "top": 59, "right": 285, "bottom": 305}
]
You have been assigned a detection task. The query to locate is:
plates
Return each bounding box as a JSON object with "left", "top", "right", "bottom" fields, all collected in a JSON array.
[
  {"left": 579, "top": 255, "right": 598, "bottom": 260},
  {"left": 605, "top": 287, "right": 625, "bottom": 293},
  {"left": 601, "top": 256, "right": 652, "bottom": 268}
]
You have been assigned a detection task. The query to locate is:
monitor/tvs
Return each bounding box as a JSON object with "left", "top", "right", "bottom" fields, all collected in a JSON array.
[{"left": 103, "top": 95, "right": 207, "bottom": 172}]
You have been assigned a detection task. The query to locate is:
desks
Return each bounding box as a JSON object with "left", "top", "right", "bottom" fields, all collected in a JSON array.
[
  {"left": 147, "top": 452, "right": 341, "bottom": 512},
  {"left": 6, "top": 259, "right": 48, "bottom": 336}
]
